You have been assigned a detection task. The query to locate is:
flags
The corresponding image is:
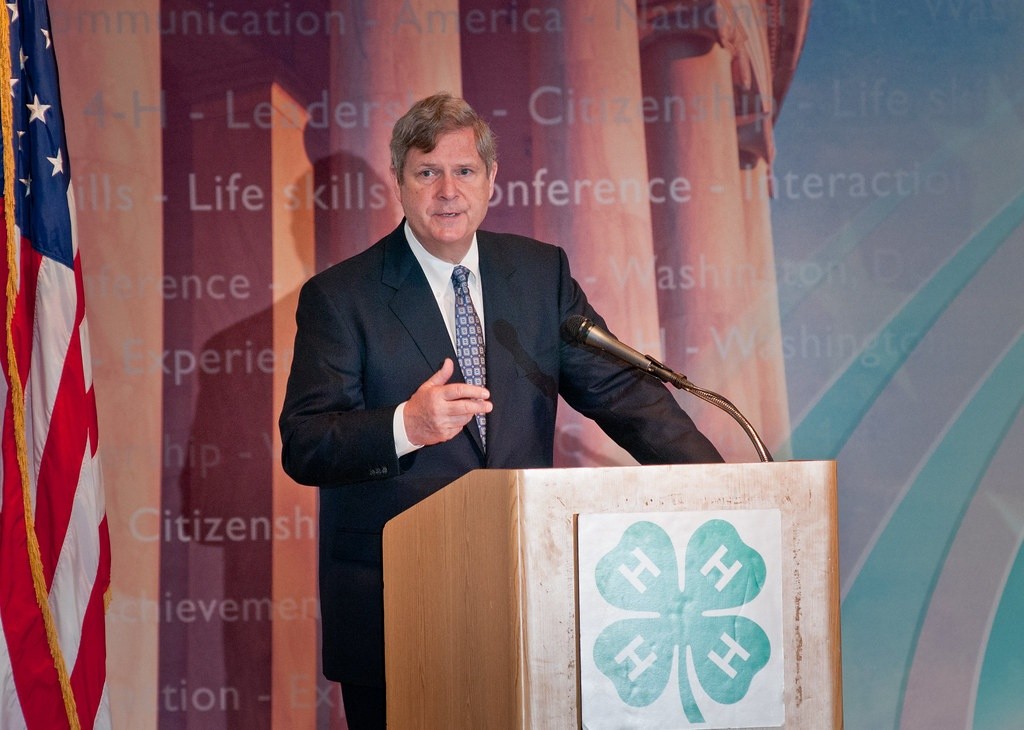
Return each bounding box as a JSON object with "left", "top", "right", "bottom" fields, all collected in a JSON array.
[{"left": 0, "top": 0, "right": 113, "bottom": 729}]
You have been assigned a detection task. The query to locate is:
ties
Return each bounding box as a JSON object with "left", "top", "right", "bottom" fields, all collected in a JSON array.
[{"left": 450, "top": 264, "right": 487, "bottom": 456}]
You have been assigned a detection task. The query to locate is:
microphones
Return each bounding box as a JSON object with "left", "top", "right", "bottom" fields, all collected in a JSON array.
[{"left": 564, "top": 315, "right": 696, "bottom": 392}]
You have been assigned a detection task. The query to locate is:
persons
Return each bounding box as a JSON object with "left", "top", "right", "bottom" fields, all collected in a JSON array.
[{"left": 278, "top": 92, "right": 727, "bottom": 730}]
